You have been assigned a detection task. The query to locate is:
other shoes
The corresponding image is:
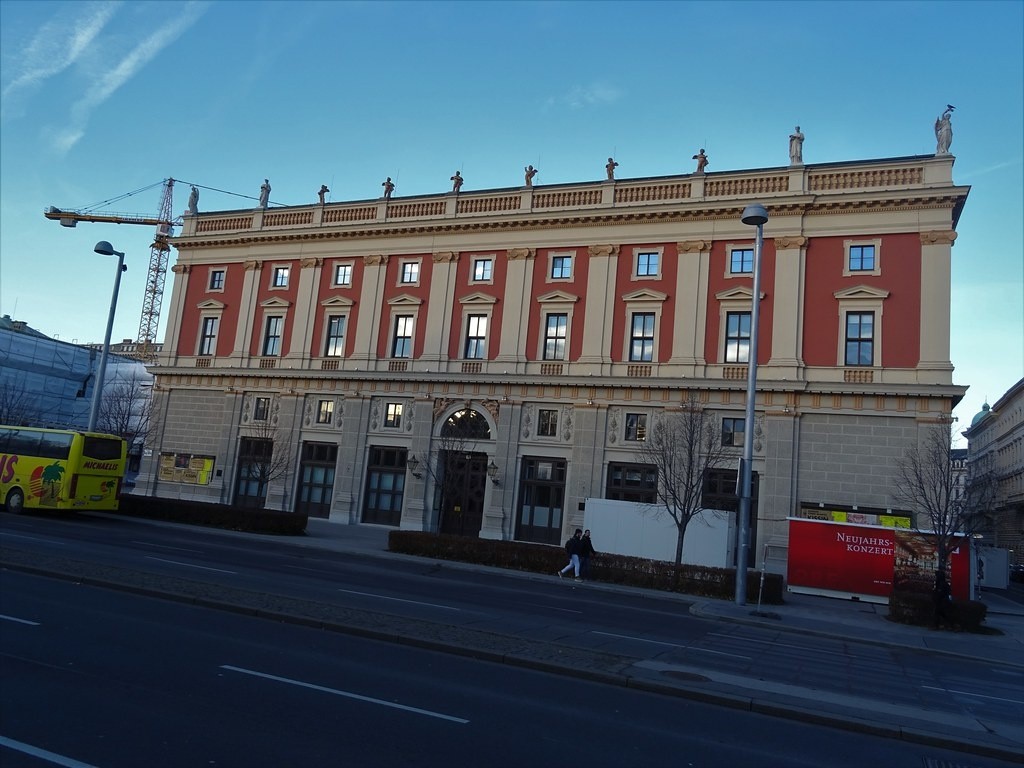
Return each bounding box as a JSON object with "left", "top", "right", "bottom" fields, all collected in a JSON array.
[
  {"left": 575, "top": 578, "right": 583, "bottom": 583},
  {"left": 558, "top": 571, "right": 563, "bottom": 579}
]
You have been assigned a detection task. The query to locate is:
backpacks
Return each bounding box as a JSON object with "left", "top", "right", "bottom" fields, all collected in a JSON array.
[{"left": 565, "top": 538, "right": 575, "bottom": 552}]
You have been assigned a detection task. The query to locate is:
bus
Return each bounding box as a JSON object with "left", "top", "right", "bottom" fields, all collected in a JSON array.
[{"left": 0, "top": 421, "right": 128, "bottom": 515}]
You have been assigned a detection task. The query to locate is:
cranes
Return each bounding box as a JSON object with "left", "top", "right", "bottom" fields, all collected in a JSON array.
[{"left": 41, "top": 177, "right": 287, "bottom": 365}]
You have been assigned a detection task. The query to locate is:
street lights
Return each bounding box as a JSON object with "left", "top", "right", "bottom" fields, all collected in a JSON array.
[
  {"left": 87, "top": 241, "right": 129, "bottom": 432},
  {"left": 734, "top": 201, "right": 767, "bottom": 607}
]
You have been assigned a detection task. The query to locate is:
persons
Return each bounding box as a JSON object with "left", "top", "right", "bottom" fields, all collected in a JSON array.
[
  {"left": 928, "top": 571, "right": 952, "bottom": 631},
  {"left": 188, "top": 187, "right": 199, "bottom": 217},
  {"left": 788, "top": 125, "right": 805, "bottom": 164},
  {"left": 525, "top": 165, "right": 538, "bottom": 187},
  {"left": 450, "top": 171, "right": 463, "bottom": 192},
  {"left": 318, "top": 185, "right": 329, "bottom": 205},
  {"left": 606, "top": 158, "right": 618, "bottom": 183},
  {"left": 934, "top": 104, "right": 956, "bottom": 155},
  {"left": 558, "top": 528, "right": 596, "bottom": 583},
  {"left": 692, "top": 149, "right": 709, "bottom": 176},
  {"left": 260, "top": 179, "right": 271, "bottom": 208},
  {"left": 382, "top": 177, "right": 394, "bottom": 198}
]
